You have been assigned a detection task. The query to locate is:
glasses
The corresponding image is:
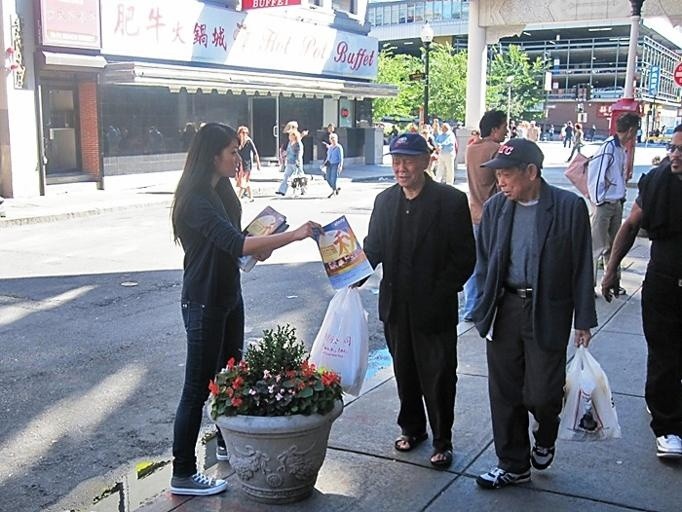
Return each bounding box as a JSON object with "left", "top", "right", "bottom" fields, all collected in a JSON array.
[{"left": 665, "top": 143, "right": 682, "bottom": 154}]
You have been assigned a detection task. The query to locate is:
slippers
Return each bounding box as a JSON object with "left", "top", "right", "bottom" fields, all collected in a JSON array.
[
  {"left": 430, "top": 450, "right": 452, "bottom": 466},
  {"left": 395, "top": 432, "right": 428, "bottom": 452}
]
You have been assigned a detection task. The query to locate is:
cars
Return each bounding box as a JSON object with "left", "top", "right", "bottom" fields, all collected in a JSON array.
[
  {"left": 662, "top": 126, "right": 676, "bottom": 145},
  {"left": 645, "top": 132, "right": 672, "bottom": 145},
  {"left": 570, "top": 82, "right": 624, "bottom": 99}
]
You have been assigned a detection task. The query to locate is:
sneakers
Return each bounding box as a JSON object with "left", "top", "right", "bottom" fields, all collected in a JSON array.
[
  {"left": 610, "top": 287, "right": 625, "bottom": 295},
  {"left": 656, "top": 435, "right": 682, "bottom": 457},
  {"left": 476, "top": 467, "right": 531, "bottom": 489},
  {"left": 170, "top": 473, "right": 228, "bottom": 496},
  {"left": 530, "top": 441, "right": 557, "bottom": 472},
  {"left": 217, "top": 446, "right": 228, "bottom": 461}
]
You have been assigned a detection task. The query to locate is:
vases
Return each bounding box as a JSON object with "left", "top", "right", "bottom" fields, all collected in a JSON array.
[{"left": 207, "top": 396, "right": 348, "bottom": 502}]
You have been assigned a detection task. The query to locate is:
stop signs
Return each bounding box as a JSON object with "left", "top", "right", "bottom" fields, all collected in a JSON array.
[{"left": 674, "top": 62, "right": 682, "bottom": 89}]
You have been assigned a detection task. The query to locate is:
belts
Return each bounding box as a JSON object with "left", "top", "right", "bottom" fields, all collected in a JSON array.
[{"left": 517, "top": 288, "right": 533, "bottom": 299}]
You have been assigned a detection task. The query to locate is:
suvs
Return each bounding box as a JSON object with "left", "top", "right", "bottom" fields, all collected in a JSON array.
[{"left": 383, "top": 113, "right": 453, "bottom": 145}]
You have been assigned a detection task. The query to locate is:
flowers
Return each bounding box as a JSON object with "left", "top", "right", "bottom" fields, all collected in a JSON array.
[{"left": 206, "top": 321, "right": 346, "bottom": 419}]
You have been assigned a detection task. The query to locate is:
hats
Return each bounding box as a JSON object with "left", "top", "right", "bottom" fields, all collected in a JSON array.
[
  {"left": 480, "top": 139, "right": 543, "bottom": 169},
  {"left": 382, "top": 134, "right": 427, "bottom": 157}
]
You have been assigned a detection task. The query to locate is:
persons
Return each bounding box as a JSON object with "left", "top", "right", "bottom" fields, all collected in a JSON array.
[
  {"left": 348, "top": 128, "right": 479, "bottom": 473},
  {"left": 509, "top": 119, "right": 540, "bottom": 143},
  {"left": 168, "top": 121, "right": 325, "bottom": 494},
  {"left": 462, "top": 107, "right": 510, "bottom": 325},
  {"left": 321, "top": 124, "right": 343, "bottom": 199},
  {"left": 584, "top": 110, "right": 643, "bottom": 301},
  {"left": 599, "top": 121, "right": 682, "bottom": 463},
  {"left": 550, "top": 120, "right": 596, "bottom": 161},
  {"left": 391, "top": 118, "right": 457, "bottom": 185},
  {"left": 466, "top": 135, "right": 599, "bottom": 491},
  {"left": 103, "top": 120, "right": 199, "bottom": 156},
  {"left": 234, "top": 125, "right": 261, "bottom": 203},
  {"left": 274, "top": 121, "right": 306, "bottom": 197}
]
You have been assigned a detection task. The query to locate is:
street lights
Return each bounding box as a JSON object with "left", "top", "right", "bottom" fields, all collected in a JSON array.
[
  {"left": 505, "top": 75, "right": 515, "bottom": 138},
  {"left": 420, "top": 18, "right": 434, "bottom": 126}
]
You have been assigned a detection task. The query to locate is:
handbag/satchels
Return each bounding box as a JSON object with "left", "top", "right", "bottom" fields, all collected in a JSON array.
[{"left": 564, "top": 153, "right": 610, "bottom": 200}]
